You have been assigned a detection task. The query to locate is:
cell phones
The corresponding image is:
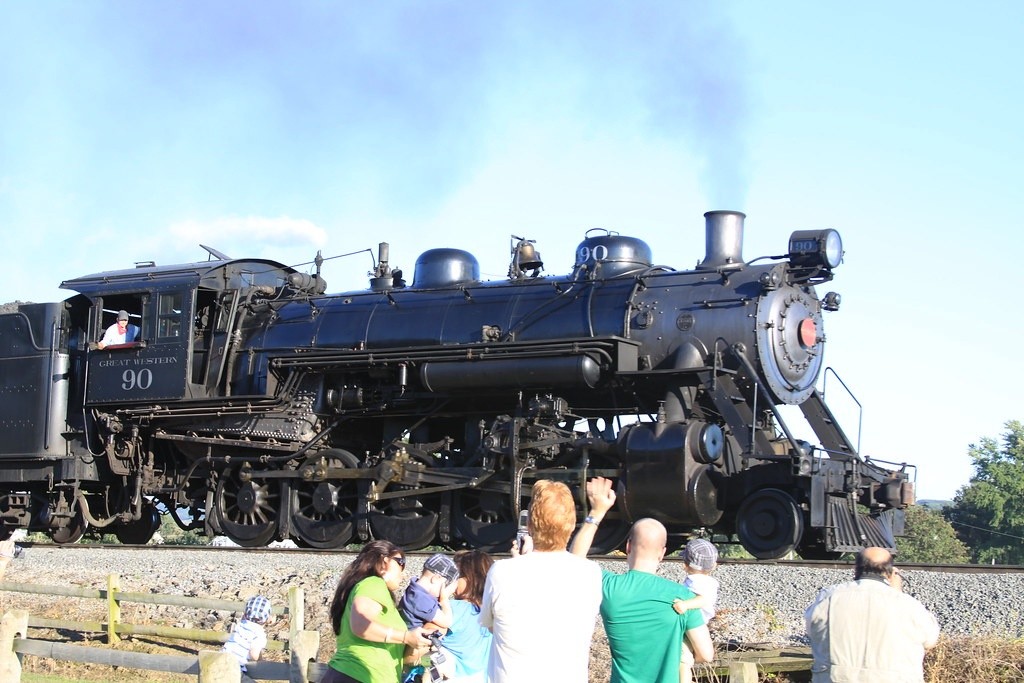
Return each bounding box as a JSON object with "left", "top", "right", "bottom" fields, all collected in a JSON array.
[
  {"left": 517, "top": 510, "right": 529, "bottom": 556},
  {"left": 429, "top": 667, "right": 442, "bottom": 683}
]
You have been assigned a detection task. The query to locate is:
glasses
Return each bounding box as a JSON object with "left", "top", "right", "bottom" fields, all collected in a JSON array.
[{"left": 383, "top": 555, "right": 406, "bottom": 571}]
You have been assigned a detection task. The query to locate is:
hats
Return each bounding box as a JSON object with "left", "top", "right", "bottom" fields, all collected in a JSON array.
[
  {"left": 679, "top": 538, "right": 718, "bottom": 571},
  {"left": 423, "top": 552, "right": 460, "bottom": 580},
  {"left": 244, "top": 597, "right": 271, "bottom": 624}
]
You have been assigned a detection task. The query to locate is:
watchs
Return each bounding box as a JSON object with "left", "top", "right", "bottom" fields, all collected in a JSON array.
[{"left": 584, "top": 516, "right": 600, "bottom": 526}]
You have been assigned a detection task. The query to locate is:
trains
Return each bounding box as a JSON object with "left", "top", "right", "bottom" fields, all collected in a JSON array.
[{"left": 0, "top": 209, "right": 919, "bottom": 561}]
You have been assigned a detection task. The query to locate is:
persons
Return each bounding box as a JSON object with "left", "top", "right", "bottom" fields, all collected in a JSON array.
[
  {"left": 321, "top": 539, "right": 432, "bottom": 683},
  {"left": 477, "top": 478, "right": 603, "bottom": 683},
  {"left": 672, "top": 538, "right": 719, "bottom": 683},
  {"left": 397, "top": 552, "right": 459, "bottom": 633},
  {"left": 803, "top": 546, "right": 940, "bottom": 683},
  {"left": 404, "top": 548, "right": 495, "bottom": 683},
  {"left": 219, "top": 596, "right": 270, "bottom": 683},
  {"left": 97, "top": 310, "right": 140, "bottom": 350},
  {"left": 569, "top": 477, "right": 715, "bottom": 683}
]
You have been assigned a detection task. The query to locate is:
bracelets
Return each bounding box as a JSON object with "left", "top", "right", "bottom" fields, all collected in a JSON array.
[
  {"left": 385, "top": 628, "right": 393, "bottom": 642},
  {"left": 402, "top": 631, "right": 407, "bottom": 643}
]
